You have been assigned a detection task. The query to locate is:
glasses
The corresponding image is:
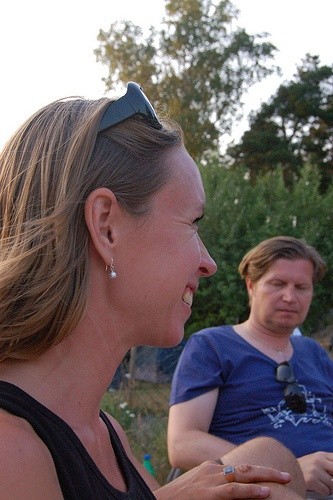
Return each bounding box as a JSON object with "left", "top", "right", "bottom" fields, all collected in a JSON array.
[
  {"left": 98, "top": 81, "right": 163, "bottom": 132},
  {"left": 275, "top": 360, "right": 306, "bottom": 414}
]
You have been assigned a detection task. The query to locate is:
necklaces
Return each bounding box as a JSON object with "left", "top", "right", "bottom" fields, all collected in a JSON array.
[{"left": 241, "top": 324, "right": 290, "bottom": 359}]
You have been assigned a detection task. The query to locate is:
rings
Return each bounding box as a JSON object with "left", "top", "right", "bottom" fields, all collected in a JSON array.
[{"left": 222, "top": 466, "right": 235, "bottom": 482}]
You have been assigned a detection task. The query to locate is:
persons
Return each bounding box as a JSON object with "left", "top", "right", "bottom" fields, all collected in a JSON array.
[
  {"left": 0, "top": 80, "right": 306, "bottom": 500},
  {"left": 166, "top": 236, "right": 333, "bottom": 500}
]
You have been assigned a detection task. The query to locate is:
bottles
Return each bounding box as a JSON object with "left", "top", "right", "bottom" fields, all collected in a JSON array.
[{"left": 143, "top": 454, "right": 156, "bottom": 479}]
